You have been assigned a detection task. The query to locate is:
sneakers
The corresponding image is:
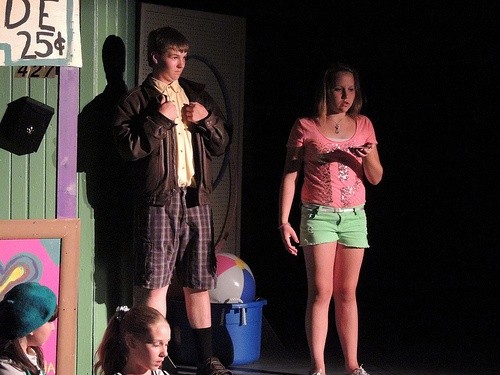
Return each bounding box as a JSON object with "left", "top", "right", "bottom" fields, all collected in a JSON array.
[
  {"left": 196, "top": 357, "right": 233, "bottom": 375},
  {"left": 343, "top": 364, "right": 370, "bottom": 375}
]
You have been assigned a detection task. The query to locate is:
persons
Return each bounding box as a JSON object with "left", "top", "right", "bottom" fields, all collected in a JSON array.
[
  {"left": 0, "top": 282, "right": 59, "bottom": 375},
  {"left": 93, "top": 306, "right": 171, "bottom": 375},
  {"left": 279, "top": 65, "right": 383, "bottom": 375},
  {"left": 112, "top": 26, "right": 233, "bottom": 374}
]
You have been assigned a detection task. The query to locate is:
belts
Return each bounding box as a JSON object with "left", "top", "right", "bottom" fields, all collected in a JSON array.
[{"left": 303, "top": 205, "right": 365, "bottom": 212}]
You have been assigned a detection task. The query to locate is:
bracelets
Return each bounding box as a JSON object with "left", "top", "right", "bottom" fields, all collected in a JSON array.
[{"left": 279, "top": 223, "right": 291, "bottom": 229}]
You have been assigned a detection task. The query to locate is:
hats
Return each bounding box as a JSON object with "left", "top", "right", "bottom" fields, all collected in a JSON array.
[{"left": 0, "top": 282, "right": 57, "bottom": 338}]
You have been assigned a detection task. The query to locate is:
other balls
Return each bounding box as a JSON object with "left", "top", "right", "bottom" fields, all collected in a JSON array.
[{"left": 200, "top": 252, "right": 258, "bottom": 305}]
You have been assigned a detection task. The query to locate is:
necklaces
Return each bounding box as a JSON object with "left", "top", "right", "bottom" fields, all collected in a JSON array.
[{"left": 326, "top": 116, "right": 344, "bottom": 134}]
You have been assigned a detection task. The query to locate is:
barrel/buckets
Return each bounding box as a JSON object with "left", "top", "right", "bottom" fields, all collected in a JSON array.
[{"left": 211, "top": 298, "right": 268, "bottom": 366}]
